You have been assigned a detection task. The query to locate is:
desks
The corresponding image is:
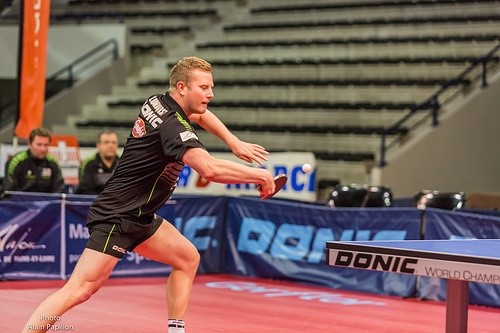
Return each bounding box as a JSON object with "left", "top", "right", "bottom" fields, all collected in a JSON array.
[{"left": 325, "top": 239, "right": 500, "bottom": 333}]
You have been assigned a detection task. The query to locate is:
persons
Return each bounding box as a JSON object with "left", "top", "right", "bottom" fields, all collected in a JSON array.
[
  {"left": 5, "top": 128, "right": 68, "bottom": 193},
  {"left": 78, "top": 128, "right": 122, "bottom": 195},
  {"left": 19, "top": 56, "right": 277, "bottom": 333}
]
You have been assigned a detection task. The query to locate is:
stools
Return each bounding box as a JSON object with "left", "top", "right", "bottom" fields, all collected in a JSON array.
[{"left": 51, "top": 0, "right": 500, "bottom": 163}]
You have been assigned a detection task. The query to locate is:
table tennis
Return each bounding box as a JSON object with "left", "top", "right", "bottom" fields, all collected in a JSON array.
[{"left": 303, "top": 164, "right": 311, "bottom": 172}]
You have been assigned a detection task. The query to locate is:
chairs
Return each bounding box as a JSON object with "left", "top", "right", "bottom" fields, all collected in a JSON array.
[
  {"left": 412, "top": 190, "right": 466, "bottom": 210},
  {"left": 327, "top": 183, "right": 392, "bottom": 208}
]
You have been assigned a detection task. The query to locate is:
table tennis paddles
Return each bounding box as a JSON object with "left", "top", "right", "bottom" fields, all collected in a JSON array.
[{"left": 256, "top": 174, "right": 288, "bottom": 198}]
